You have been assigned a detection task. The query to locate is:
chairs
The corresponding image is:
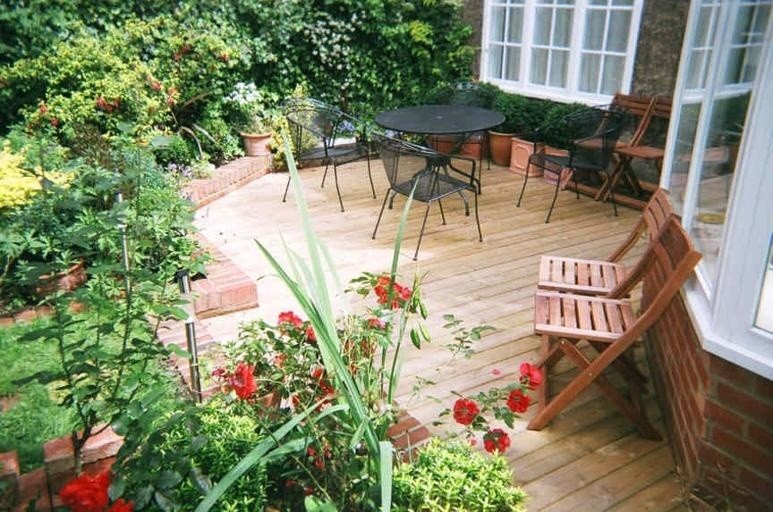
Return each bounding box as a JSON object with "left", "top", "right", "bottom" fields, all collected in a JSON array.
[{"left": 277, "top": 96, "right": 483, "bottom": 262}]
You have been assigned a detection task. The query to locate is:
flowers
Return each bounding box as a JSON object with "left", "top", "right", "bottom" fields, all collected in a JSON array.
[
  {"left": 275, "top": 270, "right": 546, "bottom": 501},
  {"left": 226, "top": 76, "right": 277, "bottom": 130},
  {"left": 0, "top": 142, "right": 95, "bottom": 269},
  {"left": 56, "top": 466, "right": 137, "bottom": 512}
]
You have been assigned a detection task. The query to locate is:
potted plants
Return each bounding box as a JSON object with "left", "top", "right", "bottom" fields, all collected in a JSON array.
[
  {"left": 221, "top": 317, "right": 285, "bottom": 421},
  {"left": 421, "top": 81, "right": 602, "bottom": 191}
]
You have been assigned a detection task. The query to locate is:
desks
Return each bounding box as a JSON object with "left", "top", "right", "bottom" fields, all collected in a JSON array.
[{"left": 374, "top": 104, "right": 508, "bottom": 217}]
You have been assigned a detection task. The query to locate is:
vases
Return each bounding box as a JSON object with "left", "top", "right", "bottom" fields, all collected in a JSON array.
[
  {"left": 21, "top": 254, "right": 87, "bottom": 302},
  {"left": 239, "top": 124, "right": 273, "bottom": 158}
]
